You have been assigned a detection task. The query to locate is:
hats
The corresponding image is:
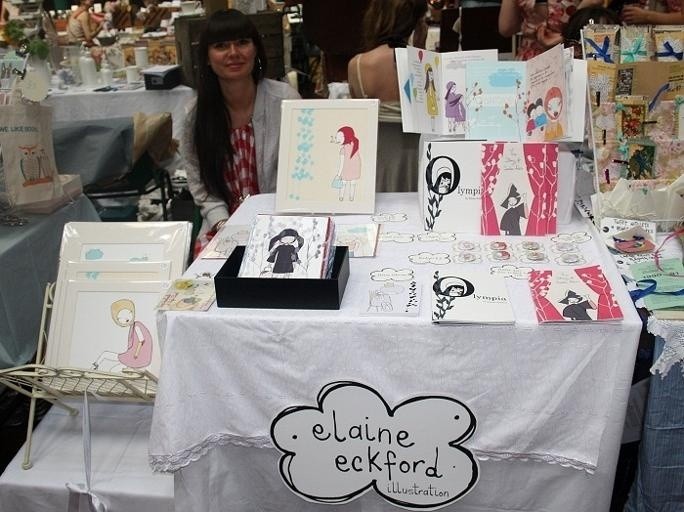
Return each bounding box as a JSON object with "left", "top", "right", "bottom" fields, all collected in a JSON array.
[
  {"left": 424, "top": 62, "right": 432, "bottom": 73},
  {"left": 446, "top": 81, "right": 455, "bottom": 90}
]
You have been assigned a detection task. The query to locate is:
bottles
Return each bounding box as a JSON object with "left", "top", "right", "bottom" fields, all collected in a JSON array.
[{"left": 78, "top": 49, "right": 112, "bottom": 87}]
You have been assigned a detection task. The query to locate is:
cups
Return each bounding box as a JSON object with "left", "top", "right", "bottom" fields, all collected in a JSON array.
[{"left": 126, "top": 47, "right": 149, "bottom": 84}]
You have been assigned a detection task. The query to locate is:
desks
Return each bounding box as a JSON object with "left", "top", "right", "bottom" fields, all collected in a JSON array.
[{"left": 0, "top": 71, "right": 684, "bottom": 512}]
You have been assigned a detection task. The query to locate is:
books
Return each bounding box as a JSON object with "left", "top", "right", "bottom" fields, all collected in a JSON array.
[
  {"left": 151, "top": 46, "right": 684, "bottom": 327},
  {"left": 43, "top": 220, "right": 194, "bottom": 395}
]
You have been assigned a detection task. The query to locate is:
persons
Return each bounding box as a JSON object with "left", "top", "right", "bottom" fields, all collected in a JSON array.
[
  {"left": 178, "top": 8, "right": 302, "bottom": 263},
  {"left": 65, "top": 1, "right": 105, "bottom": 48},
  {"left": 282, "top": 0, "right": 684, "bottom": 86},
  {"left": 347, "top": 1, "right": 435, "bottom": 192}
]
